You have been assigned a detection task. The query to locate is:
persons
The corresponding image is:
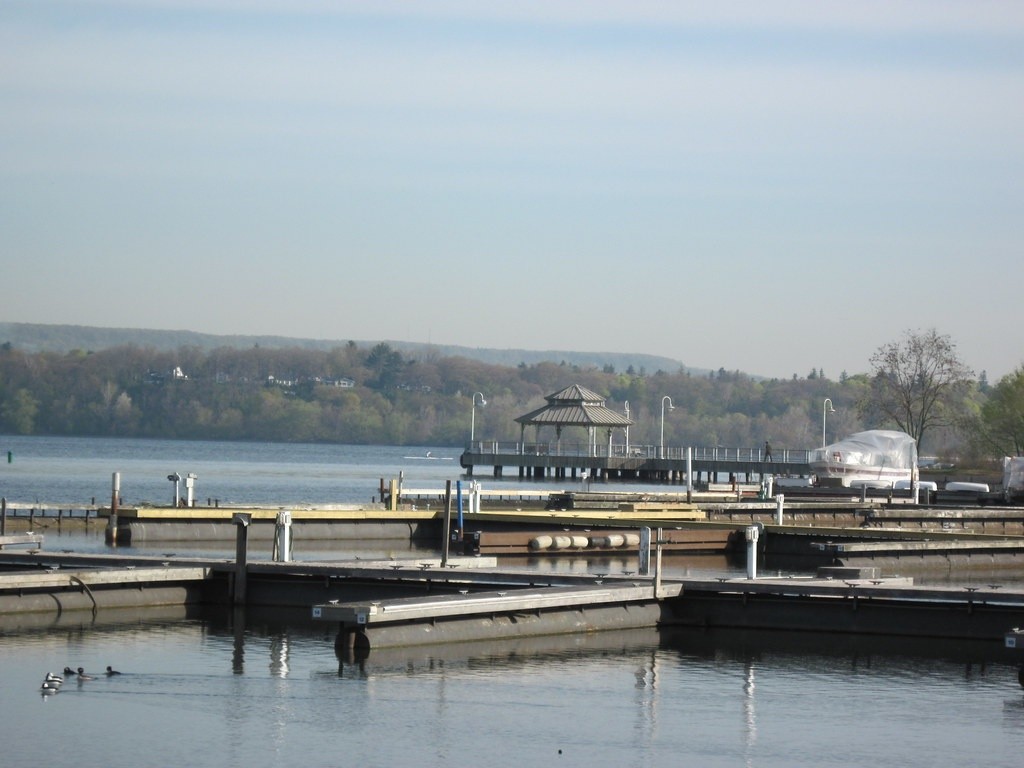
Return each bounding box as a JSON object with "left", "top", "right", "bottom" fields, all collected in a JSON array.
[{"left": 763, "top": 442, "right": 773, "bottom": 463}]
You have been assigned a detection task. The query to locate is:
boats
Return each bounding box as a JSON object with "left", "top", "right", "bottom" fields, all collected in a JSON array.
[
  {"left": 809, "top": 429, "right": 919, "bottom": 487},
  {"left": 403, "top": 456, "right": 454, "bottom": 459}
]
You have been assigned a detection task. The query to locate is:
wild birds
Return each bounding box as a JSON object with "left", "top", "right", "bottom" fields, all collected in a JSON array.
[{"left": 40, "top": 665, "right": 122, "bottom": 696}]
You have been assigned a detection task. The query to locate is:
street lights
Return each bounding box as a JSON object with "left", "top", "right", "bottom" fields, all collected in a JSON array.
[
  {"left": 623, "top": 400, "right": 630, "bottom": 454},
  {"left": 472, "top": 392, "right": 487, "bottom": 441},
  {"left": 822, "top": 398, "right": 835, "bottom": 447},
  {"left": 661, "top": 396, "right": 675, "bottom": 459}
]
